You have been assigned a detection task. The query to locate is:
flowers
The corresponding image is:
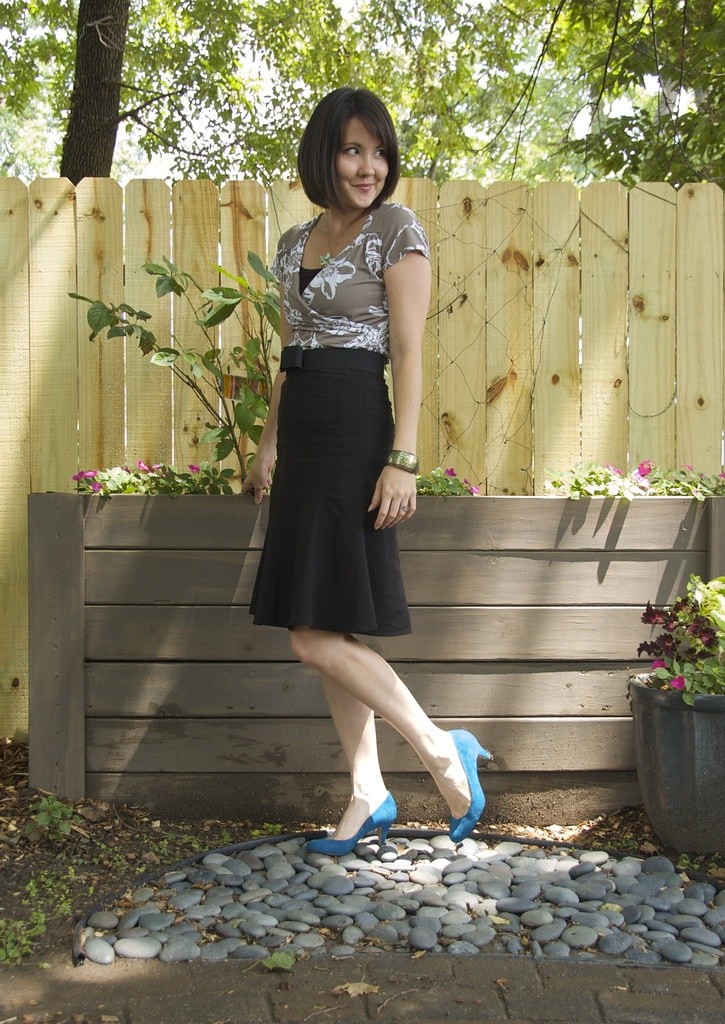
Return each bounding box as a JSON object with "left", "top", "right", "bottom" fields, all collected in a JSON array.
[
  {"left": 634, "top": 574, "right": 725, "bottom": 708},
  {"left": 70, "top": 250, "right": 725, "bottom": 503}
]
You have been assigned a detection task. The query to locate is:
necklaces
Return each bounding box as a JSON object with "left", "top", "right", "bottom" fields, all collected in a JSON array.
[{"left": 319, "top": 213, "right": 357, "bottom": 268}]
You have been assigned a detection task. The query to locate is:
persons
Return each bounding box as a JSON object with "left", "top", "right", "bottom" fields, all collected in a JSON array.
[{"left": 241, "top": 87, "right": 492, "bottom": 855}]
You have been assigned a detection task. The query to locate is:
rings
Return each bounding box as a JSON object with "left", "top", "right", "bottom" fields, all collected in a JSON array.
[{"left": 400, "top": 506, "right": 409, "bottom": 511}]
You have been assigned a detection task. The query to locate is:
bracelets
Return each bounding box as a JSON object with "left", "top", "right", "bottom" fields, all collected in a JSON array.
[{"left": 384, "top": 450, "right": 418, "bottom": 475}]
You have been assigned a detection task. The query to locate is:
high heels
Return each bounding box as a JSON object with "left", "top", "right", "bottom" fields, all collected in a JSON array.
[
  {"left": 306, "top": 790, "right": 397, "bottom": 856},
  {"left": 447, "top": 729, "right": 493, "bottom": 843}
]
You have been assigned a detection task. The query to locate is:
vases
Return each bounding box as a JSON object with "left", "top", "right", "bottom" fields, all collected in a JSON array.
[{"left": 628, "top": 673, "right": 725, "bottom": 856}]
